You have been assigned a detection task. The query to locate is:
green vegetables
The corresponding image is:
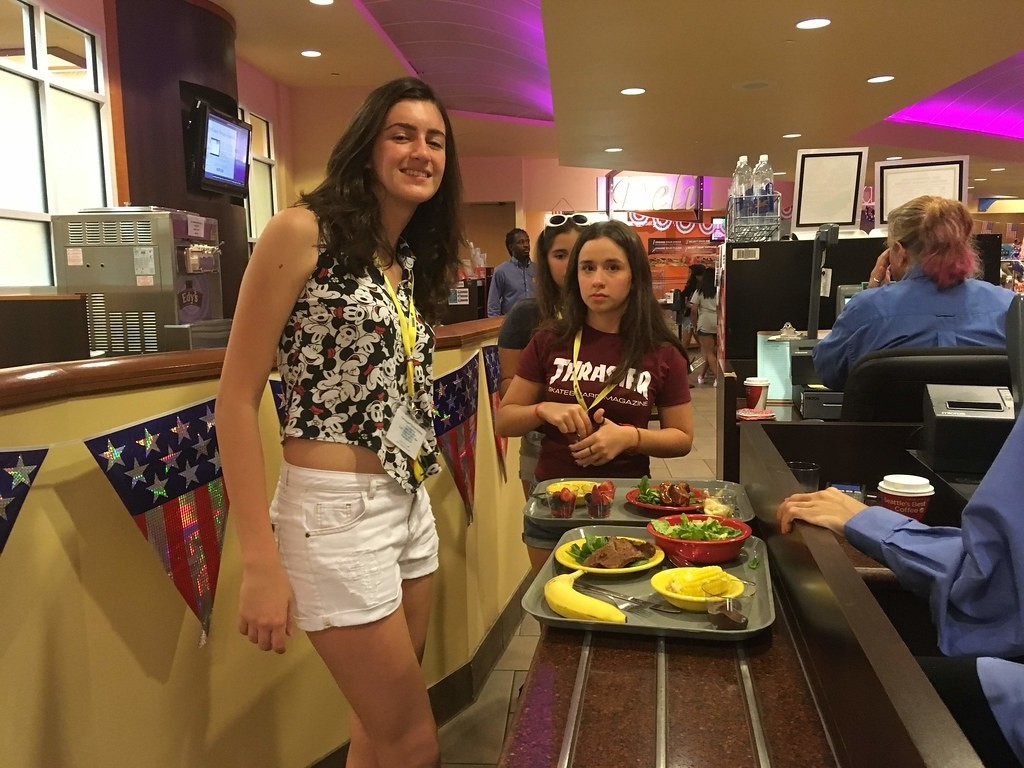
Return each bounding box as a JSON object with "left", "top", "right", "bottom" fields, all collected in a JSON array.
[
  {"left": 749, "top": 557, "right": 760, "bottom": 569},
  {"left": 565, "top": 534, "right": 608, "bottom": 563},
  {"left": 630, "top": 475, "right": 702, "bottom": 506},
  {"left": 650, "top": 513, "right": 742, "bottom": 541}
]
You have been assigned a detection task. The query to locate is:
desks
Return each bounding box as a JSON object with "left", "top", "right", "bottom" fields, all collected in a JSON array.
[{"left": 660, "top": 299, "right": 693, "bottom": 341}]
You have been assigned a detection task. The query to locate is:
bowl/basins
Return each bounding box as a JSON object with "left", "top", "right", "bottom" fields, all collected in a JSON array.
[
  {"left": 546, "top": 481, "right": 599, "bottom": 507},
  {"left": 647, "top": 514, "right": 752, "bottom": 564},
  {"left": 650, "top": 568, "right": 744, "bottom": 613}
]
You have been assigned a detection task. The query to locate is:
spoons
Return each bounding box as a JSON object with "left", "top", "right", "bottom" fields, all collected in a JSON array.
[{"left": 668, "top": 555, "right": 756, "bottom": 585}]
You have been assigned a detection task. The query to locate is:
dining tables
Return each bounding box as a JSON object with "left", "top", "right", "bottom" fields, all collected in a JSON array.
[{"left": 495, "top": 580, "right": 848, "bottom": 768}]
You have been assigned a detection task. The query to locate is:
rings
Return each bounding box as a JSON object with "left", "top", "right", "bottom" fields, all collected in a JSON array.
[
  {"left": 594, "top": 416, "right": 605, "bottom": 426},
  {"left": 872, "top": 277, "right": 879, "bottom": 283},
  {"left": 587, "top": 445, "right": 595, "bottom": 454}
]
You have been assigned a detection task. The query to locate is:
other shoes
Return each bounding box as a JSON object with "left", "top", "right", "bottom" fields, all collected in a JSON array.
[
  {"left": 713, "top": 379, "right": 717, "bottom": 388},
  {"left": 698, "top": 375, "right": 705, "bottom": 384}
]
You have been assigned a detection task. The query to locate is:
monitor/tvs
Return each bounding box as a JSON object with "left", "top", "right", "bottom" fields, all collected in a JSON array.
[
  {"left": 188, "top": 100, "right": 253, "bottom": 198},
  {"left": 710, "top": 216, "right": 725, "bottom": 242}
]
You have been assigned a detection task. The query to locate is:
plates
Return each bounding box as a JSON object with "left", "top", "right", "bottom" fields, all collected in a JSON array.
[
  {"left": 626, "top": 486, "right": 705, "bottom": 511},
  {"left": 555, "top": 536, "right": 665, "bottom": 575}
]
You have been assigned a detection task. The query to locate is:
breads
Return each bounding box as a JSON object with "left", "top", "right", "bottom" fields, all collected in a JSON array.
[{"left": 584, "top": 535, "right": 656, "bottom": 568}]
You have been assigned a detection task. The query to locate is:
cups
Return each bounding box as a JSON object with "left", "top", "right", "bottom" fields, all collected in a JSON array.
[
  {"left": 877, "top": 475, "right": 935, "bottom": 522},
  {"left": 702, "top": 488, "right": 736, "bottom": 518},
  {"left": 743, "top": 377, "right": 770, "bottom": 411},
  {"left": 701, "top": 579, "right": 757, "bottom": 630},
  {"left": 545, "top": 484, "right": 579, "bottom": 518},
  {"left": 581, "top": 483, "right": 616, "bottom": 520},
  {"left": 787, "top": 462, "right": 821, "bottom": 494}
]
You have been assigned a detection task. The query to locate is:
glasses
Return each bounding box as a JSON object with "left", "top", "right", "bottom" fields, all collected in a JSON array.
[{"left": 544, "top": 213, "right": 590, "bottom": 236}]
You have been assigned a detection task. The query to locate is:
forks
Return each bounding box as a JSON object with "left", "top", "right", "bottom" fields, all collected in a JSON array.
[{"left": 580, "top": 590, "right": 650, "bottom": 617}]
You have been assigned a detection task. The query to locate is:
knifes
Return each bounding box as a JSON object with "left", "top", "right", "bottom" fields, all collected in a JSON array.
[{"left": 575, "top": 580, "right": 681, "bottom": 613}]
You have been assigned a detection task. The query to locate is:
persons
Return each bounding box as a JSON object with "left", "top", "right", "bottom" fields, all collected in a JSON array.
[
  {"left": 215, "top": 78, "right": 473, "bottom": 768},
  {"left": 676, "top": 263, "right": 720, "bottom": 388},
  {"left": 775, "top": 403, "right": 1024, "bottom": 768},
  {"left": 487, "top": 226, "right": 539, "bottom": 316},
  {"left": 496, "top": 208, "right": 693, "bottom": 622},
  {"left": 810, "top": 195, "right": 1024, "bottom": 390}
]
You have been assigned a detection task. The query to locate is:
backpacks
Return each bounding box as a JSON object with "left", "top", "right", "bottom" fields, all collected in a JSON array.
[{"left": 672, "top": 289, "right": 689, "bottom": 311}]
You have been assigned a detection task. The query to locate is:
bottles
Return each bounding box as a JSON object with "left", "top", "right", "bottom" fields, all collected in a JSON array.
[{"left": 729, "top": 154, "right": 774, "bottom": 224}]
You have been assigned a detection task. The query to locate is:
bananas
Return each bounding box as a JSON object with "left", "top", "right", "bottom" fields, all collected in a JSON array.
[{"left": 544, "top": 570, "right": 627, "bottom": 622}]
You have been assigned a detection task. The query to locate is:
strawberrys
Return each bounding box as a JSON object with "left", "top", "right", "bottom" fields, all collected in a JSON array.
[{"left": 550, "top": 480, "right": 616, "bottom": 517}]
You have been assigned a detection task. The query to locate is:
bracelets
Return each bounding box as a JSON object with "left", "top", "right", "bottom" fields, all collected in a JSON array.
[
  {"left": 534, "top": 401, "right": 545, "bottom": 422},
  {"left": 622, "top": 422, "right": 643, "bottom": 455}
]
